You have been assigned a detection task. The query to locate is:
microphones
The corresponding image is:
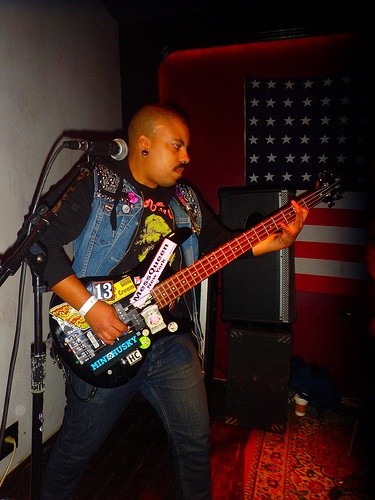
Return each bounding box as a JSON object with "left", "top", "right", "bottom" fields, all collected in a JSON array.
[{"left": 63, "top": 138, "right": 128, "bottom": 161}]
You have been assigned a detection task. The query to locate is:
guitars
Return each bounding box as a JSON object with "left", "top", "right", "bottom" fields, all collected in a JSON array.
[{"left": 49, "top": 167, "right": 346, "bottom": 389}]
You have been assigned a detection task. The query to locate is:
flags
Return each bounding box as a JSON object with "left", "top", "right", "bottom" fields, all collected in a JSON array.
[{"left": 242, "top": 78, "right": 375, "bottom": 297}]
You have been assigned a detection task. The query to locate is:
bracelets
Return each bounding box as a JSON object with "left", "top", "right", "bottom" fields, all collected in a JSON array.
[{"left": 78, "top": 296, "right": 99, "bottom": 317}]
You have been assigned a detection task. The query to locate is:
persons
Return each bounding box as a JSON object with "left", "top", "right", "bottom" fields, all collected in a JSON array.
[{"left": 25, "top": 106, "right": 309, "bottom": 500}]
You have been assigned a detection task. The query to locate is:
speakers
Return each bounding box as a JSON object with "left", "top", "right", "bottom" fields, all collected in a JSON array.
[
  {"left": 218, "top": 186, "right": 295, "bottom": 324},
  {"left": 223, "top": 325, "right": 293, "bottom": 434}
]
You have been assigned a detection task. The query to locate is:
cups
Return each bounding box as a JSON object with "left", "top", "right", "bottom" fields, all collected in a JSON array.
[{"left": 294, "top": 392, "right": 310, "bottom": 416}]
